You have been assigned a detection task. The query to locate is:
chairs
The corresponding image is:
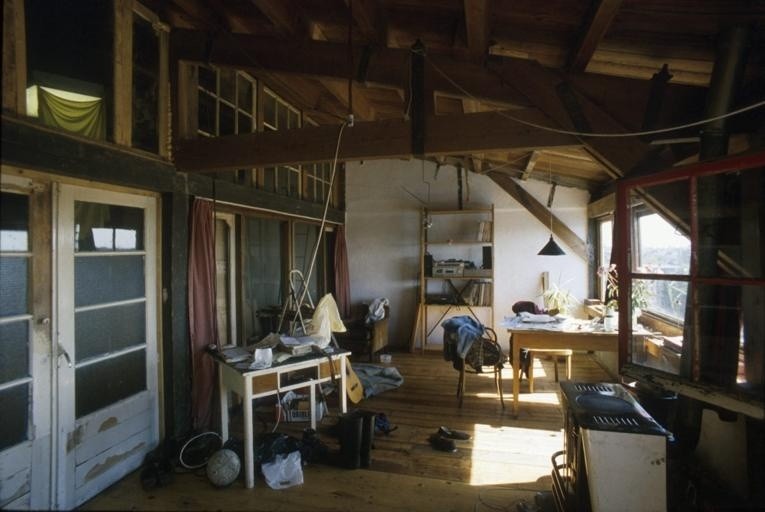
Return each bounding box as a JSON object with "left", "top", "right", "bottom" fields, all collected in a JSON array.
[
  {"left": 512, "top": 301, "right": 573, "bottom": 393},
  {"left": 452, "top": 316, "right": 505, "bottom": 410},
  {"left": 341, "top": 304, "right": 390, "bottom": 362}
]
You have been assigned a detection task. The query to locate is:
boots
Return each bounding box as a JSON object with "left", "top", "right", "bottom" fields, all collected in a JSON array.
[
  {"left": 327, "top": 413, "right": 363, "bottom": 469},
  {"left": 352, "top": 409, "right": 376, "bottom": 468}
]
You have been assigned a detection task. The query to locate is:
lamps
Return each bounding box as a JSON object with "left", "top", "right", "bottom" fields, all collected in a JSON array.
[{"left": 537, "top": 156, "right": 566, "bottom": 256}]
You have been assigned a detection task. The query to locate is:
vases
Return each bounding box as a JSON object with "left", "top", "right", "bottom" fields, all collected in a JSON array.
[{"left": 614, "top": 309, "right": 637, "bottom": 330}]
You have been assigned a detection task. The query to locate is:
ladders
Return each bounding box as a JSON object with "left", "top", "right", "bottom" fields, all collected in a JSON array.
[{"left": 274, "top": 269, "right": 350, "bottom": 416}]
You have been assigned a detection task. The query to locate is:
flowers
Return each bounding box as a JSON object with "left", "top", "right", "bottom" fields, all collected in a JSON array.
[{"left": 596, "top": 262, "right": 648, "bottom": 311}]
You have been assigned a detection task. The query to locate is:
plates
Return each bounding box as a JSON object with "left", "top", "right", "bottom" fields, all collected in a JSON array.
[{"left": 522, "top": 314, "right": 556, "bottom": 323}]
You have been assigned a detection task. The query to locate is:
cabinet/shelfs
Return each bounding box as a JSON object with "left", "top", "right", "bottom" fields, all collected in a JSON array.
[{"left": 420, "top": 201, "right": 495, "bottom": 355}]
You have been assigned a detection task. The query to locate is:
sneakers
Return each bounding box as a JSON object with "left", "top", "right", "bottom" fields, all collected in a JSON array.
[
  {"left": 438, "top": 426, "right": 471, "bottom": 440},
  {"left": 428, "top": 434, "right": 457, "bottom": 452}
]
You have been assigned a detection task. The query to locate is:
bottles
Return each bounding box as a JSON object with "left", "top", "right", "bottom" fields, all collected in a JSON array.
[{"left": 604, "top": 314, "right": 615, "bottom": 333}]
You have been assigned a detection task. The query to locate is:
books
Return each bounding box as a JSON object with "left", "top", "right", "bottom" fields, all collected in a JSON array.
[
  {"left": 466, "top": 281, "right": 492, "bottom": 306},
  {"left": 486, "top": 221, "right": 492, "bottom": 242},
  {"left": 217, "top": 347, "right": 250, "bottom": 364},
  {"left": 482, "top": 221, "right": 489, "bottom": 242},
  {"left": 475, "top": 219, "right": 485, "bottom": 242}
]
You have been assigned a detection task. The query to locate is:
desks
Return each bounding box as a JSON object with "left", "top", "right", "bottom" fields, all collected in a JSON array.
[
  {"left": 210, "top": 345, "right": 352, "bottom": 488},
  {"left": 507, "top": 315, "right": 653, "bottom": 418}
]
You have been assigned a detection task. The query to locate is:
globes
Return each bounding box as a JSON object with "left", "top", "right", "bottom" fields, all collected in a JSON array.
[{"left": 179, "top": 431, "right": 240, "bottom": 487}]
